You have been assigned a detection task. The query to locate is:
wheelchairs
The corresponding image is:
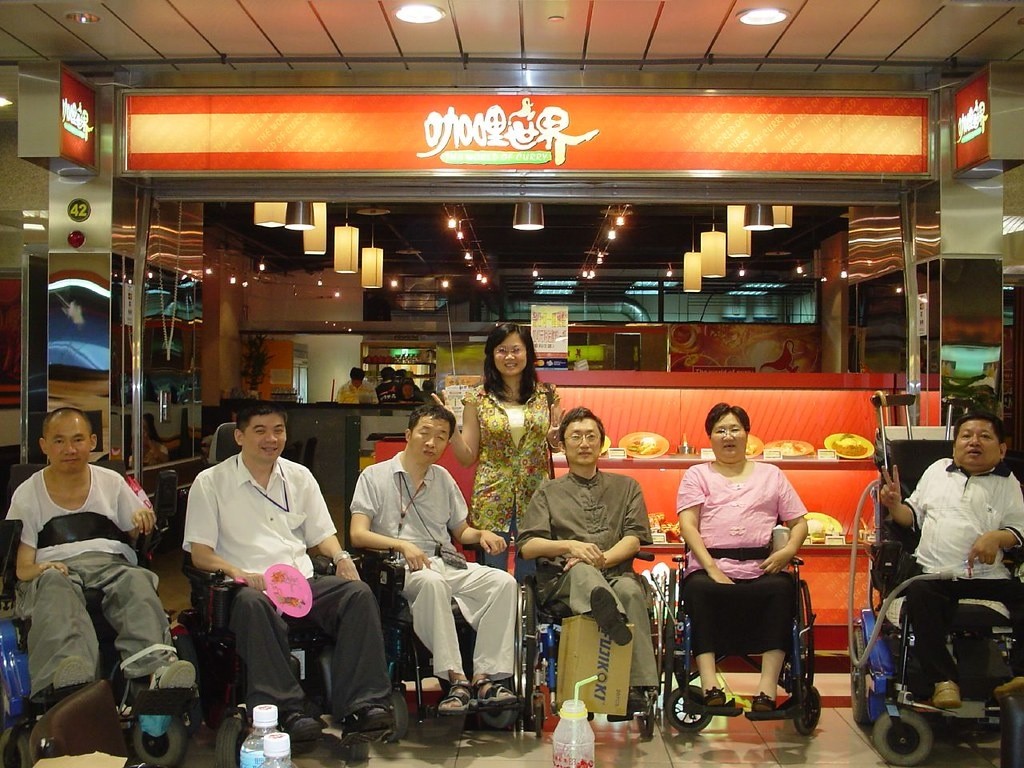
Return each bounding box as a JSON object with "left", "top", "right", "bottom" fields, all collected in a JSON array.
[
  {"left": 848, "top": 437, "right": 1024, "bottom": 768},
  {"left": 509, "top": 545, "right": 664, "bottom": 742},
  {"left": 349, "top": 541, "right": 522, "bottom": 745},
  {"left": 1, "top": 518, "right": 191, "bottom": 768},
  {"left": 174, "top": 549, "right": 395, "bottom": 768},
  {"left": 662, "top": 512, "right": 823, "bottom": 738}
]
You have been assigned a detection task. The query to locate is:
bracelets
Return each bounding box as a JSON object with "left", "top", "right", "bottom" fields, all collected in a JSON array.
[{"left": 551, "top": 444, "right": 560, "bottom": 448}]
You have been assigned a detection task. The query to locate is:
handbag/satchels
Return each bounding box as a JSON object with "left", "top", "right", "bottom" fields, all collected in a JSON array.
[{"left": 440, "top": 546, "right": 469, "bottom": 570}]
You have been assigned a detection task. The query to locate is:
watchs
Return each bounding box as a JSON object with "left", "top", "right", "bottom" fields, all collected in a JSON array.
[{"left": 333, "top": 551, "right": 350, "bottom": 564}]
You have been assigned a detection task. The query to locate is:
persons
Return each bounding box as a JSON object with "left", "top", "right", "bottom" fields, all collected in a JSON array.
[
  {"left": 430, "top": 319, "right": 567, "bottom": 586},
  {"left": 676, "top": 402, "right": 808, "bottom": 712},
  {"left": 129, "top": 412, "right": 170, "bottom": 469},
  {"left": 182, "top": 404, "right": 393, "bottom": 755},
  {"left": 5, "top": 407, "right": 196, "bottom": 736},
  {"left": 336, "top": 367, "right": 378, "bottom": 403},
  {"left": 375, "top": 366, "right": 407, "bottom": 403},
  {"left": 351, "top": 403, "right": 517, "bottom": 714},
  {"left": 516, "top": 407, "right": 658, "bottom": 712},
  {"left": 879, "top": 411, "right": 1024, "bottom": 708},
  {"left": 396, "top": 368, "right": 437, "bottom": 403}
]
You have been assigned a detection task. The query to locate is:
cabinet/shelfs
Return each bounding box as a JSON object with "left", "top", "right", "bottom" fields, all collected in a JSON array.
[
  {"left": 553, "top": 454, "right": 869, "bottom": 557},
  {"left": 360, "top": 340, "right": 436, "bottom": 379}
]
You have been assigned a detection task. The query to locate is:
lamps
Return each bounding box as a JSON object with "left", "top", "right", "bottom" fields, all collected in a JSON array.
[
  {"left": 727, "top": 205, "right": 751, "bottom": 257},
  {"left": 303, "top": 202, "right": 327, "bottom": 255},
  {"left": 390, "top": 206, "right": 630, "bottom": 290},
  {"left": 700, "top": 206, "right": 726, "bottom": 278},
  {"left": 285, "top": 202, "right": 316, "bottom": 230},
  {"left": 254, "top": 202, "right": 288, "bottom": 228},
  {"left": 361, "top": 224, "right": 383, "bottom": 288},
  {"left": 334, "top": 203, "right": 359, "bottom": 274},
  {"left": 743, "top": 204, "right": 793, "bottom": 231}
]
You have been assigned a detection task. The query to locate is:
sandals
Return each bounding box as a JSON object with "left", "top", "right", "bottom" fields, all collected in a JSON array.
[
  {"left": 438, "top": 680, "right": 473, "bottom": 713},
  {"left": 473, "top": 678, "right": 517, "bottom": 704}
]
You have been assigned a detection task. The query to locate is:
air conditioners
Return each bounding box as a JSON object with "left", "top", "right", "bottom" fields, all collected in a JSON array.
[{"left": 666, "top": 222, "right": 702, "bottom": 292}]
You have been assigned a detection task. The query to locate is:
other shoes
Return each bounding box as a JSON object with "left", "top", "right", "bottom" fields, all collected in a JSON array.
[
  {"left": 932, "top": 680, "right": 961, "bottom": 707},
  {"left": 590, "top": 586, "right": 632, "bottom": 646},
  {"left": 143, "top": 660, "right": 196, "bottom": 737},
  {"left": 341, "top": 706, "right": 394, "bottom": 738},
  {"left": 628, "top": 691, "right": 648, "bottom": 714},
  {"left": 51, "top": 654, "right": 94, "bottom": 690},
  {"left": 702, "top": 685, "right": 728, "bottom": 707},
  {"left": 752, "top": 691, "right": 776, "bottom": 711},
  {"left": 285, "top": 711, "right": 324, "bottom": 753}
]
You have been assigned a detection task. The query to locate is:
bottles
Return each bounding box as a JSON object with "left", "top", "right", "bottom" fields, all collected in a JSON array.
[
  {"left": 271, "top": 387, "right": 296, "bottom": 401},
  {"left": 299, "top": 396, "right": 303, "bottom": 404},
  {"left": 257, "top": 732, "right": 298, "bottom": 768},
  {"left": 552, "top": 699, "right": 595, "bottom": 768},
  {"left": 240, "top": 704, "right": 280, "bottom": 768}
]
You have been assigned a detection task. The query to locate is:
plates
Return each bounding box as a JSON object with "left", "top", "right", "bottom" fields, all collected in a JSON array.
[
  {"left": 764, "top": 439, "right": 814, "bottom": 457},
  {"left": 804, "top": 512, "right": 843, "bottom": 534},
  {"left": 824, "top": 433, "right": 875, "bottom": 459},
  {"left": 601, "top": 435, "right": 611, "bottom": 454},
  {"left": 745, "top": 434, "right": 764, "bottom": 458},
  {"left": 619, "top": 432, "right": 670, "bottom": 459}
]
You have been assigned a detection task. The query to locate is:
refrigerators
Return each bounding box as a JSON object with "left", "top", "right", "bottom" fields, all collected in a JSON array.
[{"left": 243, "top": 339, "right": 309, "bottom": 403}]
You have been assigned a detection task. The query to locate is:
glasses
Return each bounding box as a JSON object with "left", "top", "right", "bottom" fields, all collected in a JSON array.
[
  {"left": 497, "top": 345, "right": 523, "bottom": 354},
  {"left": 569, "top": 433, "right": 598, "bottom": 442},
  {"left": 716, "top": 427, "right": 741, "bottom": 435}
]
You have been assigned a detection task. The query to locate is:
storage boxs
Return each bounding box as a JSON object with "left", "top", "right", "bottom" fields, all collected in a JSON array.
[{"left": 556, "top": 615, "right": 634, "bottom": 716}]
